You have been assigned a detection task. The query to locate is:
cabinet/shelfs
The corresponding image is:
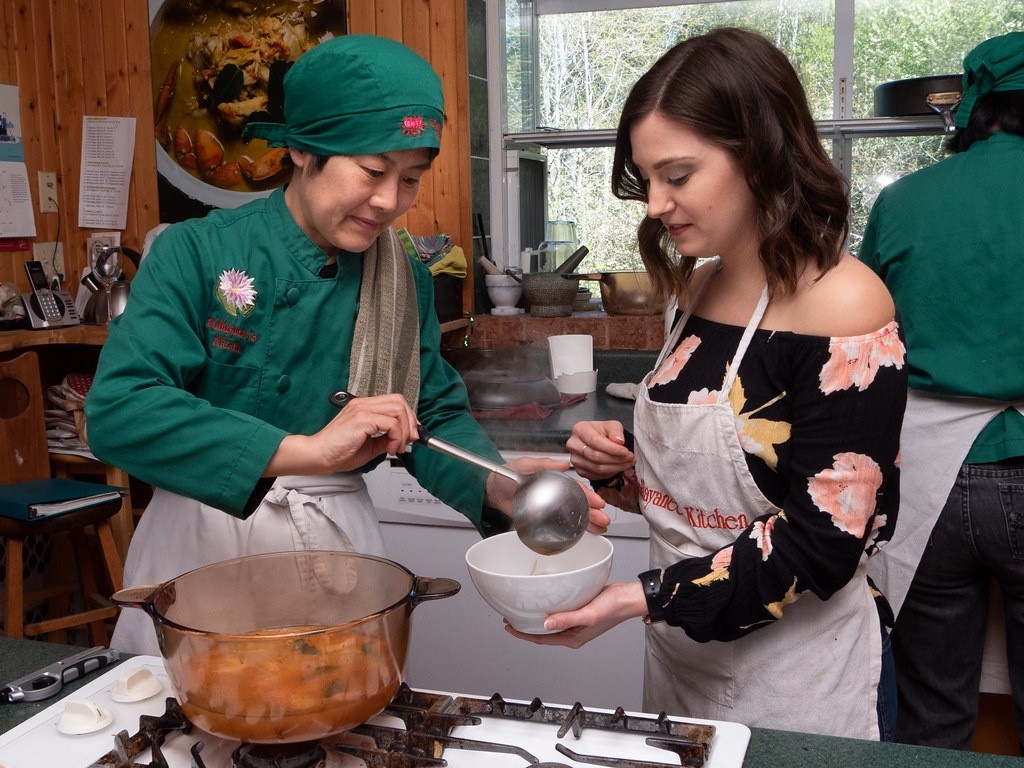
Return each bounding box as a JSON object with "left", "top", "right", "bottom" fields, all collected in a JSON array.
[{"left": 4, "top": 323, "right": 155, "bottom": 583}]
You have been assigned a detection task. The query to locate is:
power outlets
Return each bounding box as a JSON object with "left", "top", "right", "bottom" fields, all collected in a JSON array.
[{"left": 30, "top": 241, "right": 66, "bottom": 283}]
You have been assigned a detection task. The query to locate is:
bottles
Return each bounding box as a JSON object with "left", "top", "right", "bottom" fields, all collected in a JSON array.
[{"left": 538, "top": 221, "right": 578, "bottom": 271}]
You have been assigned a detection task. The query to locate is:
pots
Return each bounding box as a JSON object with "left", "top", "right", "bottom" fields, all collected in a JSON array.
[
  {"left": 111, "top": 548, "right": 460, "bottom": 743},
  {"left": 562, "top": 270, "right": 664, "bottom": 316}
]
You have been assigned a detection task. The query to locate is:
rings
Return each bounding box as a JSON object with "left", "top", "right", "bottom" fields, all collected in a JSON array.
[{"left": 582, "top": 445, "right": 587, "bottom": 456}]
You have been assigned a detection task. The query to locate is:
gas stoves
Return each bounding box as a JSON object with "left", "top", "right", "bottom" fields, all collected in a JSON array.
[{"left": 0, "top": 650, "right": 754, "bottom": 768}]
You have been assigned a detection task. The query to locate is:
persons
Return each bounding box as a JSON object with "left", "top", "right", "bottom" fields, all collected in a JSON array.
[
  {"left": 84, "top": 31, "right": 610, "bottom": 658},
  {"left": 854, "top": 33, "right": 1024, "bottom": 754},
  {"left": 498, "top": 27, "right": 908, "bottom": 740}
]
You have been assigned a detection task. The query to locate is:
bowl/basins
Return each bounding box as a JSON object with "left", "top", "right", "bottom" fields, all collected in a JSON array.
[
  {"left": 522, "top": 272, "right": 578, "bottom": 316},
  {"left": 486, "top": 275, "right": 522, "bottom": 309},
  {"left": 465, "top": 531, "right": 614, "bottom": 635},
  {"left": 460, "top": 368, "right": 563, "bottom": 411}
]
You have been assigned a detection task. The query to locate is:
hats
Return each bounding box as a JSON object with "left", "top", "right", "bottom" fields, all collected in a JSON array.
[
  {"left": 241, "top": 34, "right": 444, "bottom": 159},
  {"left": 953, "top": 32, "right": 1024, "bottom": 128}
]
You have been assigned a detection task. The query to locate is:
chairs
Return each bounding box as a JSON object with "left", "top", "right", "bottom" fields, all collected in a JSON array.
[{"left": 0, "top": 352, "right": 127, "bottom": 648}]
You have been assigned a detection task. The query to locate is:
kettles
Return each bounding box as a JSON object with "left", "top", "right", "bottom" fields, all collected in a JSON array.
[{"left": 82, "top": 246, "right": 142, "bottom": 326}]
